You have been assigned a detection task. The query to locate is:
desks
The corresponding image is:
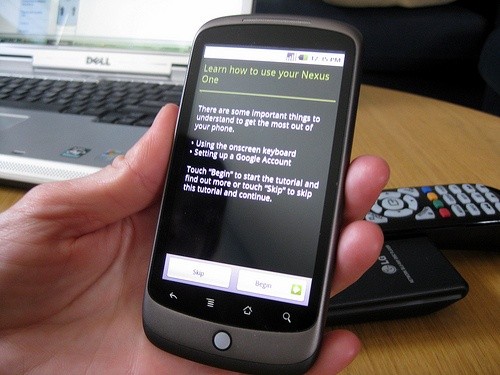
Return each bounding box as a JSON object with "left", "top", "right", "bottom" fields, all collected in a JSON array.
[{"left": 0, "top": 85, "right": 500, "bottom": 375}]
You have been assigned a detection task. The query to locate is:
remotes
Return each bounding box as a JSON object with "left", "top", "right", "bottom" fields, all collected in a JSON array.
[{"left": 364, "top": 183, "right": 500, "bottom": 240}]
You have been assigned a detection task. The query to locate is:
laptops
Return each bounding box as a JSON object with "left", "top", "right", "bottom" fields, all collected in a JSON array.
[{"left": 0, "top": 0, "right": 255, "bottom": 185}]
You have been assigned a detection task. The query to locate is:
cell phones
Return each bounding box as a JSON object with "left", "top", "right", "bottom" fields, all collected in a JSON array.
[
  {"left": 142, "top": 14, "right": 365, "bottom": 375},
  {"left": 326, "top": 236, "right": 470, "bottom": 329}
]
